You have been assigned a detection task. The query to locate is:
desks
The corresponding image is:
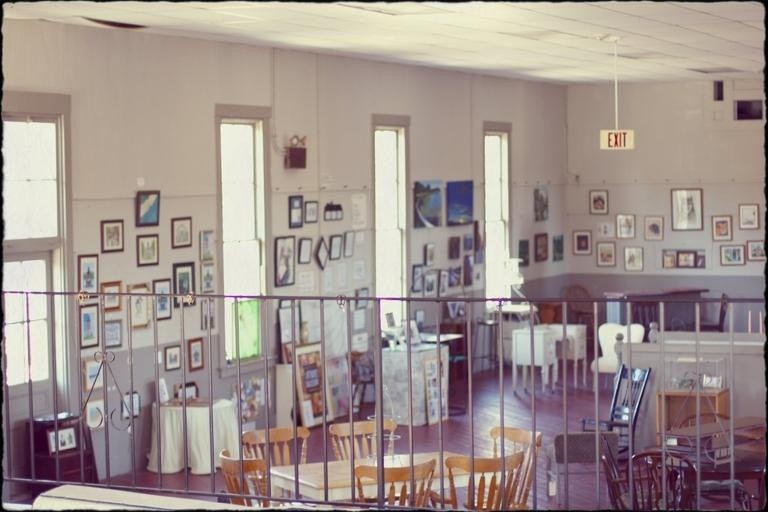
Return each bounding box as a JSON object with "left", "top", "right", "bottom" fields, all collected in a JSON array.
[
  {"left": 270, "top": 451, "right": 508, "bottom": 507},
  {"left": 147, "top": 394, "right": 240, "bottom": 476}
]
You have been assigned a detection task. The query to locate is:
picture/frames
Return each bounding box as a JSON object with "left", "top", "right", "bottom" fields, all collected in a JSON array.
[
  {"left": 271, "top": 196, "right": 370, "bottom": 430},
  {"left": 412, "top": 235, "right": 484, "bottom": 336},
  {"left": 77, "top": 193, "right": 221, "bottom": 433},
  {"left": 519, "top": 186, "right": 768, "bottom": 270}
]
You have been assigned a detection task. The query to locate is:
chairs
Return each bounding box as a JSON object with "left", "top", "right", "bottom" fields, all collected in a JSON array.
[
  {"left": 578, "top": 364, "right": 654, "bottom": 465},
  {"left": 623, "top": 406, "right": 766, "bottom": 511},
  {"left": 542, "top": 429, "right": 619, "bottom": 510},
  {"left": 328, "top": 417, "right": 399, "bottom": 460},
  {"left": 215, "top": 447, "right": 271, "bottom": 511},
  {"left": 341, "top": 285, "right": 646, "bottom": 425},
  {"left": 240, "top": 425, "right": 310, "bottom": 465},
  {"left": 487, "top": 427, "right": 545, "bottom": 508},
  {"left": 445, "top": 450, "right": 525, "bottom": 512},
  {"left": 351, "top": 458, "right": 438, "bottom": 510}
]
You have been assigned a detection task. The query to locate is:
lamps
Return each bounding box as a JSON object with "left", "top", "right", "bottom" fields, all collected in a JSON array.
[{"left": 283, "top": 135, "right": 307, "bottom": 169}]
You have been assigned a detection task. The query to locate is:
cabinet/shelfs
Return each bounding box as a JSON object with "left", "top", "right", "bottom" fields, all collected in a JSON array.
[{"left": 23, "top": 414, "right": 99, "bottom": 503}]
